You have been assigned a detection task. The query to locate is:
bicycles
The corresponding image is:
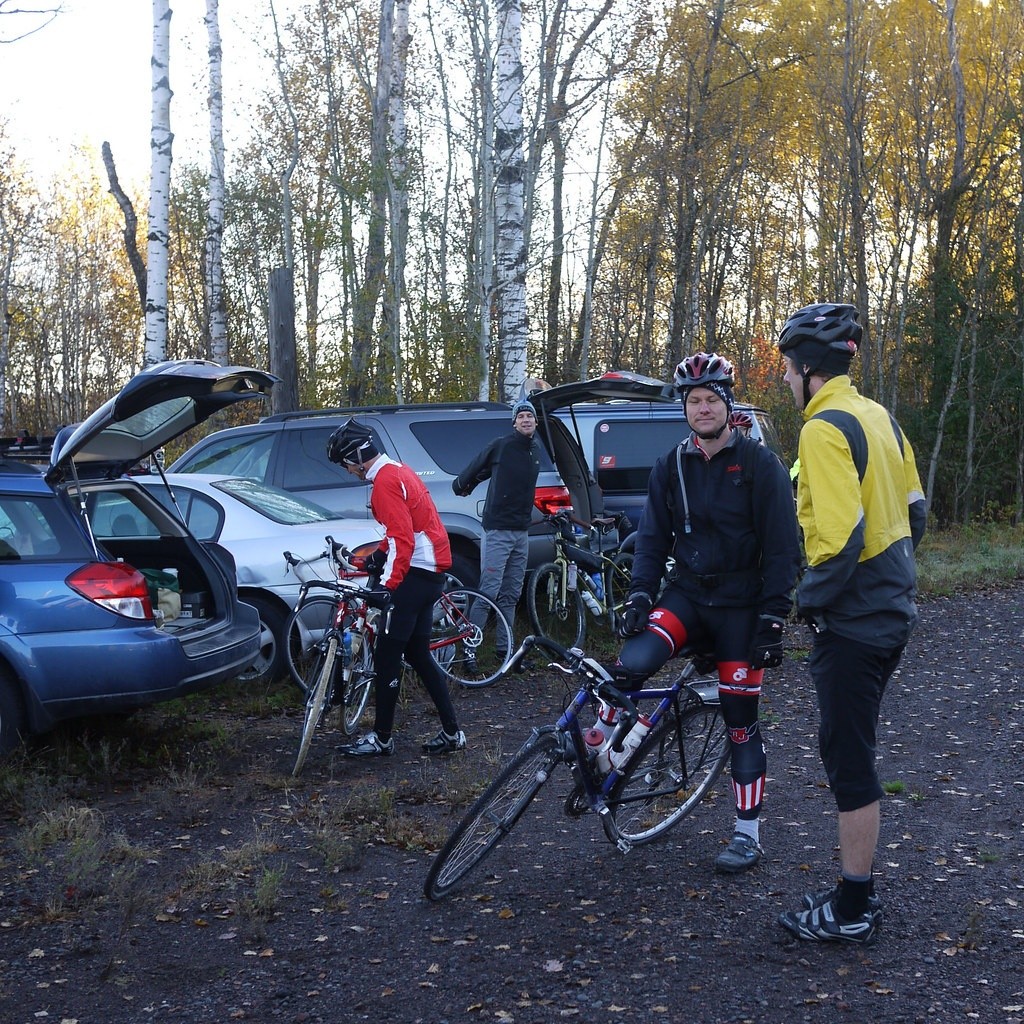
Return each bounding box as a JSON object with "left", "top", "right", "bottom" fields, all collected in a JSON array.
[
  {"left": 525, "top": 508, "right": 637, "bottom": 660},
  {"left": 420, "top": 637, "right": 737, "bottom": 901},
  {"left": 280, "top": 534, "right": 517, "bottom": 777}
]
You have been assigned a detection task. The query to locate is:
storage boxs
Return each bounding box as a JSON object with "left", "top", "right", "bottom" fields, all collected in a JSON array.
[{"left": 179, "top": 588, "right": 212, "bottom": 619}]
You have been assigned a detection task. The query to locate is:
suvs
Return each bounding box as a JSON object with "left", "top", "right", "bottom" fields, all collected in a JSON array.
[
  {"left": 451, "top": 378, "right": 797, "bottom": 556},
  {"left": 0, "top": 358, "right": 286, "bottom": 758},
  {"left": 82, "top": 368, "right": 677, "bottom": 635}
]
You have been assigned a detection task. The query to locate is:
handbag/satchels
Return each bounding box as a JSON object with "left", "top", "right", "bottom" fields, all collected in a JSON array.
[{"left": 136, "top": 568, "right": 182, "bottom": 624}]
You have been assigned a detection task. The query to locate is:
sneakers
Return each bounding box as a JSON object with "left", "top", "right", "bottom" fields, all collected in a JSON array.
[
  {"left": 495, "top": 651, "right": 526, "bottom": 675},
  {"left": 803, "top": 883, "right": 884, "bottom": 926},
  {"left": 716, "top": 833, "right": 764, "bottom": 874},
  {"left": 463, "top": 649, "right": 478, "bottom": 673},
  {"left": 422, "top": 727, "right": 467, "bottom": 754},
  {"left": 335, "top": 732, "right": 394, "bottom": 759},
  {"left": 778, "top": 899, "right": 875, "bottom": 946}
]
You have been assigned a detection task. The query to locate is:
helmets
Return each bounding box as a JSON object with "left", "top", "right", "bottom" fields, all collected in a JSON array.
[
  {"left": 779, "top": 304, "right": 863, "bottom": 351},
  {"left": 326, "top": 418, "right": 372, "bottom": 463},
  {"left": 728, "top": 412, "right": 753, "bottom": 430}
]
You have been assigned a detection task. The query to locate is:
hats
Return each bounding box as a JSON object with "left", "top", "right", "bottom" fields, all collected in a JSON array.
[
  {"left": 673, "top": 353, "right": 735, "bottom": 392},
  {"left": 681, "top": 381, "right": 734, "bottom": 411},
  {"left": 339, "top": 442, "right": 378, "bottom": 464},
  {"left": 785, "top": 340, "right": 855, "bottom": 376},
  {"left": 512, "top": 401, "right": 538, "bottom": 426}
]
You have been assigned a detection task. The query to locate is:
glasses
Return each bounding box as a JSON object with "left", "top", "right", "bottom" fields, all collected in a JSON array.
[{"left": 339, "top": 465, "right": 350, "bottom": 471}]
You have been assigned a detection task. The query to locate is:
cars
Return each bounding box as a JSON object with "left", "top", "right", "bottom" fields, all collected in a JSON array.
[{"left": 0, "top": 470, "right": 388, "bottom": 692}]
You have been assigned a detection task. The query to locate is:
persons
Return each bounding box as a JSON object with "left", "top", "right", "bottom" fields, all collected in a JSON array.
[
  {"left": 779, "top": 303, "right": 927, "bottom": 946},
  {"left": 729, "top": 411, "right": 753, "bottom": 437},
  {"left": 326, "top": 416, "right": 466, "bottom": 756},
  {"left": 588, "top": 354, "right": 798, "bottom": 875},
  {"left": 452, "top": 399, "right": 541, "bottom": 676}
]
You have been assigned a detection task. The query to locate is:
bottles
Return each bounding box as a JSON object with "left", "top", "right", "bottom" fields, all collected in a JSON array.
[
  {"left": 583, "top": 729, "right": 612, "bottom": 776},
  {"left": 610, "top": 713, "right": 652, "bottom": 770},
  {"left": 591, "top": 571, "right": 604, "bottom": 601},
  {"left": 567, "top": 561, "right": 578, "bottom": 592},
  {"left": 343, "top": 632, "right": 353, "bottom": 666},
  {"left": 349, "top": 627, "right": 362, "bottom": 654},
  {"left": 582, "top": 590, "right": 602, "bottom": 616},
  {"left": 578, "top": 569, "right": 598, "bottom": 591}
]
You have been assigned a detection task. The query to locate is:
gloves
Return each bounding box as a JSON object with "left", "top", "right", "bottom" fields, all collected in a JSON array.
[
  {"left": 748, "top": 614, "right": 785, "bottom": 670},
  {"left": 616, "top": 591, "right": 653, "bottom": 639},
  {"left": 365, "top": 549, "right": 387, "bottom": 574},
  {"left": 797, "top": 599, "right": 827, "bottom": 634},
  {"left": 366, "top": 585, "right": 392, "bottom": 610}
]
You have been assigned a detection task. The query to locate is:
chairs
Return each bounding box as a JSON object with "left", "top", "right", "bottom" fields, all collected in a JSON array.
[{"left": 111, "top": 514, "right": 140, "bottom": 536}]
